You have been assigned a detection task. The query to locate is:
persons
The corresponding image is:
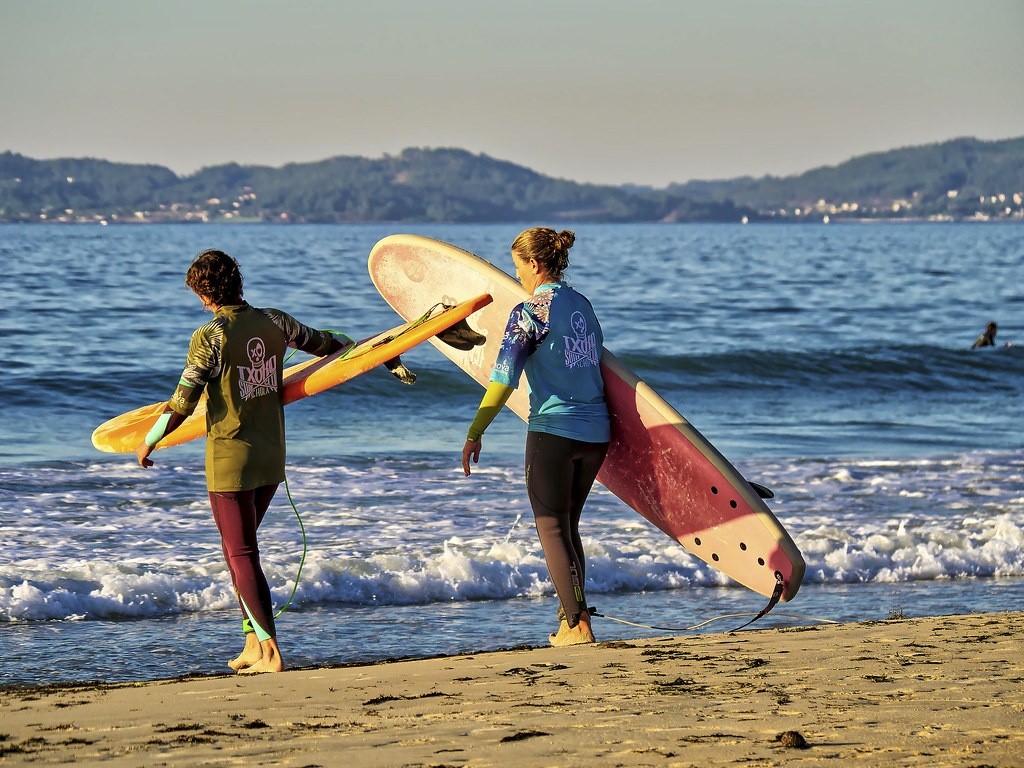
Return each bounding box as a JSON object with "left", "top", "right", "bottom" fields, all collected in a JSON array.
[
  {"left": 969, "top": 321, "right": 1008, "bottom": 351},
  {"left": 462, "top": 227, "right": 610, "bottom": 647},
  {"left": 136, "top": 249, "right": 356, "bottom": 674}
]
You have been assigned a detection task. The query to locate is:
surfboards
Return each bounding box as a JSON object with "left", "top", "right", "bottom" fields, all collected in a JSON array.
[
  {"left": 90, "top": 294, "right": 499, "bottom": 454},
  {"left": 366, "top": 234, "right": 806, "bottom": 602}
]
